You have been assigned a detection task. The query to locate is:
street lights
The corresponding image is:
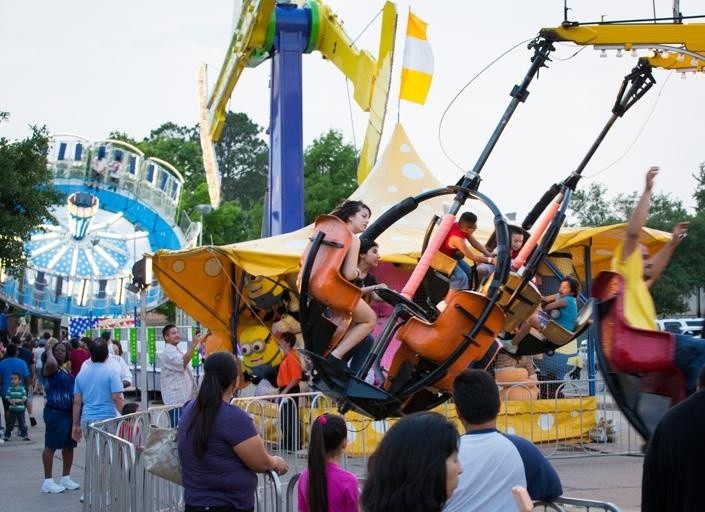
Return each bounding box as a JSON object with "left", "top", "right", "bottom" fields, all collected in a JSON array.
[
  {"left": 126, "top": 255, "right": 153, "bottom": 413},
  {"left": 196, "top": 202, "right": 213, "bottom": 244}
]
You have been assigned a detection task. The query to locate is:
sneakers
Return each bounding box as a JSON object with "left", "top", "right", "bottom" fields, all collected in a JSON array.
[
  {"left": 498, "top": 338, "right": 520, "bottom": 355},
  {"left": 272, "top": 445, "right": 293, "bottom": 455},
  {"left": 41, "top": 477, "right": 66, "bottom": 495},
  {"left": 59, "top": 473, "right": 82, "bottom": 491}
]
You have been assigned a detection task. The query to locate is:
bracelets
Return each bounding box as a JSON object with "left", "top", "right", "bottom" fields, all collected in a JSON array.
[
  {"left": 487, "top": 256, "right": 492, "bottom": 264},
  {"left": 354, "top": 270, "right": 359, "bottom": 278}
]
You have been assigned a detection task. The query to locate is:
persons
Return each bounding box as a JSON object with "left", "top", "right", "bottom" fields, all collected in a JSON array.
[
  {"left": 324, "top": 199, "right": 378, "bottom": 375},
  {"left": 0, "top": 306, "right": 142, "bottom": 508},
  {"left": 160, "top": 324, "right": 201, "bottom": 428},
  {"left": 359, "top": 411, "right": 464, "bottom": 512},
  {"left": 435, "top": 212, "right": 497, "bottom": 313},
  {"left": 439, "top": 368, "right": 562, "bottom": 512},
  {"left": 87, "top": 153, "right": 107, "bottom": 193},
  {"left": 610, "top": 167, "right": 705, "bottom": 396},
  {"left": 498, "top": 277, "right": 578, "bottom": 355},
  {"left": 108, "top": 157, "right": 122, "bottom": 192},
  {"left": 640, "top": 366, "right": 705, "bottom": 512},
  {"left": 476, "top": 229, "right": 529, "bottom": 286},
  {"left": 176, "top": 352, "right": 290, "bottom": 512},
  {"left": 297, "top": 413, "right": 360, "bottom": 512},
  {"left": 310, "top": 239, "right": 398, "bottom": 377},
  {"left": 272, "top": 332, "right": 302, "bottom": 452}
]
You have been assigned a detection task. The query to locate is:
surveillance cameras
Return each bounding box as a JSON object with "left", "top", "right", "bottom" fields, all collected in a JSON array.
[{"left": 125, "top": 282, "right": 139, "bottom": 293}]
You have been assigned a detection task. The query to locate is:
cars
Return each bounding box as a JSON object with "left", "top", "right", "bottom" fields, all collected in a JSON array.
[{"left": 576, "top": 317, "right": 703, "bottom": 363}]
